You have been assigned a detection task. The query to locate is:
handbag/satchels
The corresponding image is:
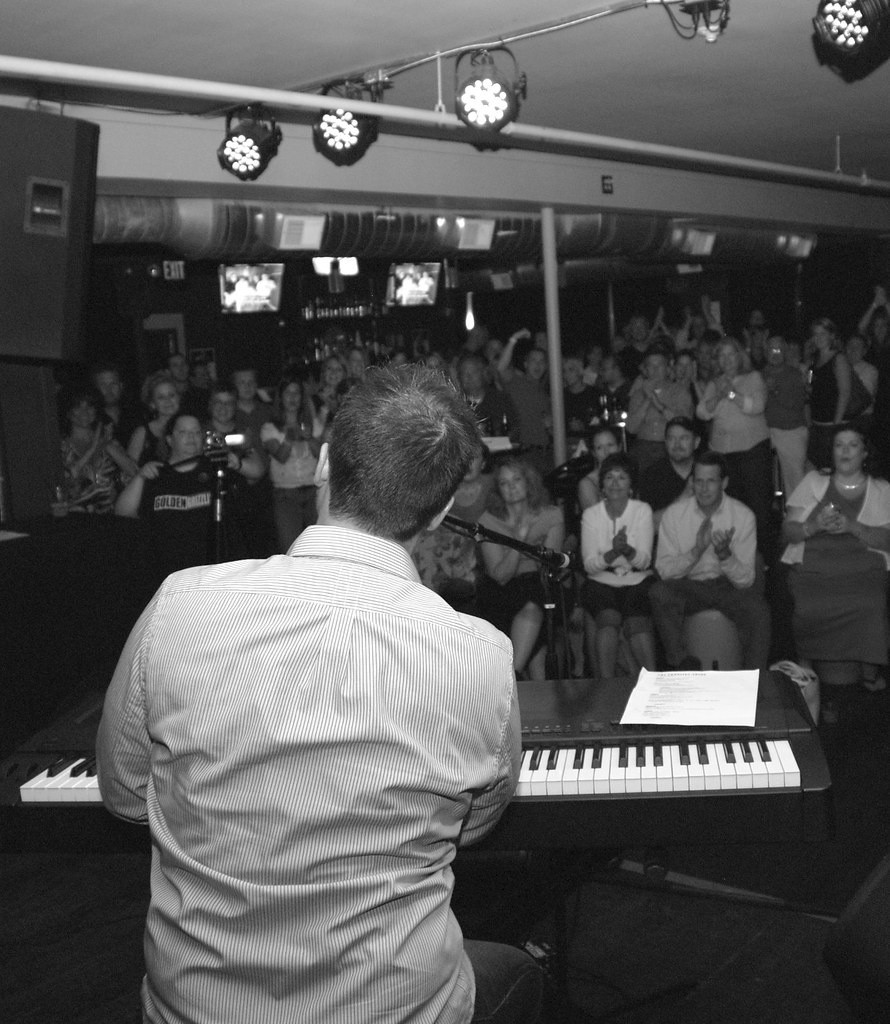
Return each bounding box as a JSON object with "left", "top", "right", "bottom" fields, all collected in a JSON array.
[{"left": 833, "top": 351, "right": 872, "bottom": 421}]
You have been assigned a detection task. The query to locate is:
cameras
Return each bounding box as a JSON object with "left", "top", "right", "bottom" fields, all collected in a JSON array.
[{"left": 202, "top": 429, "right": 245, "bottom": 450}]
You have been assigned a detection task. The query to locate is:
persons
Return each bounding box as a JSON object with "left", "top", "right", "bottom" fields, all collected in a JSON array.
[
  {"left": 32, "top": 261, "right": 890, "bottom": 725},
  {"left": 95, "top": 366, "right": 543, "bottom": 1024}
]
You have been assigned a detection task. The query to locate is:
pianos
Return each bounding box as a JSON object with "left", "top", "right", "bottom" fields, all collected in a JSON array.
[{"left": 0, "top": 661, "right": 840, "bottom": 837}]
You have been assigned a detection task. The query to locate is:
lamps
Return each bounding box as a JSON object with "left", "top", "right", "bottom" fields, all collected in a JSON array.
[
  {"left": 313, "top": 69, "right": 394, "bottom": 168},
  {"left": 214, "top": 104, "right": 284, "bottom": 181},
  {"left": 812, "top": 0, "right": 890, "bottom": 84},
  {"left": 453, "top": 45, "right": 528, "bottom": 143}
]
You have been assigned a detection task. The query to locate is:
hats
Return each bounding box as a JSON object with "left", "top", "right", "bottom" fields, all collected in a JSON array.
[{"left": 139, "top": 368, "right": 172, "bottom": 405}]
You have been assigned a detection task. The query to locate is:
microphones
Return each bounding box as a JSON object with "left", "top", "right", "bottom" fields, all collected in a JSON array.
[{"left": 541, "top": 453, "right": 598, "bottom": 501}]
